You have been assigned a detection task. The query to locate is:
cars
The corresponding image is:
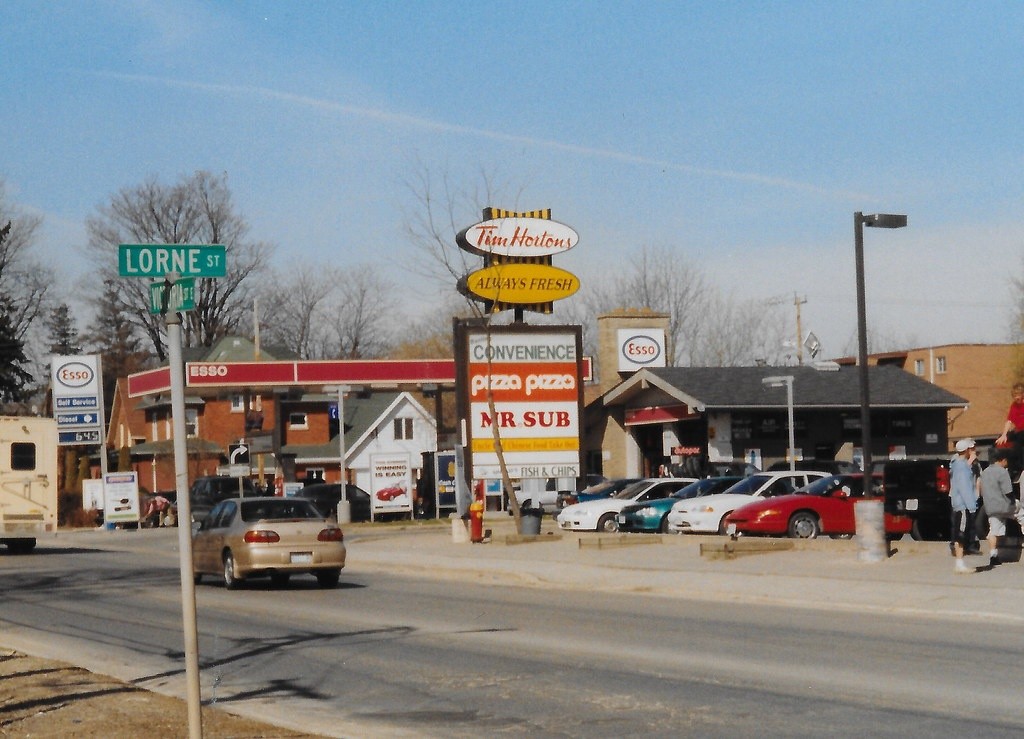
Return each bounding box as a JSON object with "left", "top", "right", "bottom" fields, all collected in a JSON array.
[
  {"left": 286, "top": 483, "right": 382, "bottom": 523},
  {"left": 556, "top": 459, "right": 911, "bottom": 540},
  {"left": 191, "top": 497, "right": 346, "bottom": 589}
]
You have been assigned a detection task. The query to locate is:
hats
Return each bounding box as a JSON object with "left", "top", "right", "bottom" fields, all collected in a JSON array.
[{"left": 956, "top": 438, "right": 977, "bottom": 452}]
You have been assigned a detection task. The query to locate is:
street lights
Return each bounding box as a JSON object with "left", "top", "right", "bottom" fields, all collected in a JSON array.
[
  {"left": 837, "top": 211, "right": 908, "bottom": 499},
  {"left": 761, "top": 376, "right": 795, "bottom": 489}
]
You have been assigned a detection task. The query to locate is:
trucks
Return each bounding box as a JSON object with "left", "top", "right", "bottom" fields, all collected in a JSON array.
[
  {"left": 883, "top": 432, "right": 1024, "bottom": 545},
  {"left": 0, "top": 412, "right": 58, "bottom": 555}
]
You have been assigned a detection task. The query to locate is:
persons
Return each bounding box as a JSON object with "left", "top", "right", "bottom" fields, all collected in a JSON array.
[
  {"left": 996, "top": 383, "right": 1024, "bottom": 470},
  {"left": 948, "top": 438, "right": 983, "bottom": 557},
  {"left": 145, "top": 496, "right": 170, "bottom": 528},
  {"left": 979, "top": 449, "right": 1024, "bottom": 565},
  {"left": 263, "top": 479, "right": 275, "bottom": 496},
  {"left": 950, "top": 441, "right": 977, "bottom": 574},
  {"left": 255, "top": 483, "right": 263, "bottom": 496}
]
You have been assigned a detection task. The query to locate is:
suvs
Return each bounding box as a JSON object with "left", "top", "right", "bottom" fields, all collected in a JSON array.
[
  {"left": 507, "top": 472, "right": 608, "bottom": 517},
  {"left": 171, "top": 475, "right": 264, "bottom": 530}
]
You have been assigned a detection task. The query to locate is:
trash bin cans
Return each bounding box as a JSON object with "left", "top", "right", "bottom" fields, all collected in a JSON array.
[{"left": 520, "top": 499, "right": 544, "bottom": 535}]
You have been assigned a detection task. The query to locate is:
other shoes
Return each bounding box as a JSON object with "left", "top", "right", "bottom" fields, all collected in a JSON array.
[
  {"left": 990, "top": 555, "right": 1003, "bottom": 567},
  {"left": 953, "top": 566, "right": 977, "bottom": 574}
]
